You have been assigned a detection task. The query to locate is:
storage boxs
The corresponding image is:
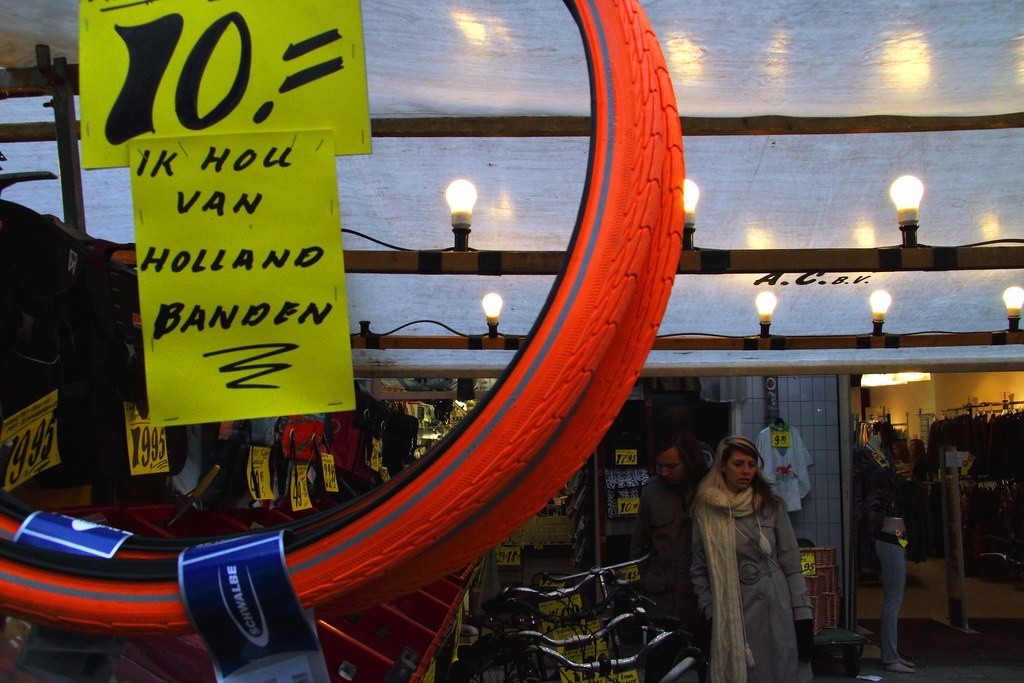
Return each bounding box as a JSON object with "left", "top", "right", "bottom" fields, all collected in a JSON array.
[{"left": 0, "top": 490, "right": 482, "bottom": 683}]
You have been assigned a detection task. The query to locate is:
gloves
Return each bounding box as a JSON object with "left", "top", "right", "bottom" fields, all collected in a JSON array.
[{"left": 795, "top": 619, "right": 814, "bottom": 663}]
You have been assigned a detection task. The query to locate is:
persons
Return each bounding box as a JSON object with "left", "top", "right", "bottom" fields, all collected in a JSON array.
[
  {"left": 858, "top": 434, "right": 897, "bottom": 583},
  {"left": 875, "top": 516, "right": 916, "bottom": 674},
  {"left": 630, "top": 425, "right": 711, "bottom": 683},
  {"left": 891, "top": 438, "right": 929, "bottom": 481},
  {"left": 685, "top": 435, "right": 815, "bottom": 683}
]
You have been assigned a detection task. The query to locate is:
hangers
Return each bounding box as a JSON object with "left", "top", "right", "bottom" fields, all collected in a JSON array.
[
  {"left": 866, "top": 415, "right": 878, "bottom": 424},
  {"left": 774, "top": 415, "right": 786, "bottom": 427},
  {"left": 934, "top": 401, "right": 1017, "bottom": 423},
  {"left": 959, "top": 478, "right": 1016, "bottom": 490}
]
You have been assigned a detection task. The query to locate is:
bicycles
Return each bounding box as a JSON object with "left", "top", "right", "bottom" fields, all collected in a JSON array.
[{"left": 442, "top": 544, "right": 701, "bottom": 683}]
